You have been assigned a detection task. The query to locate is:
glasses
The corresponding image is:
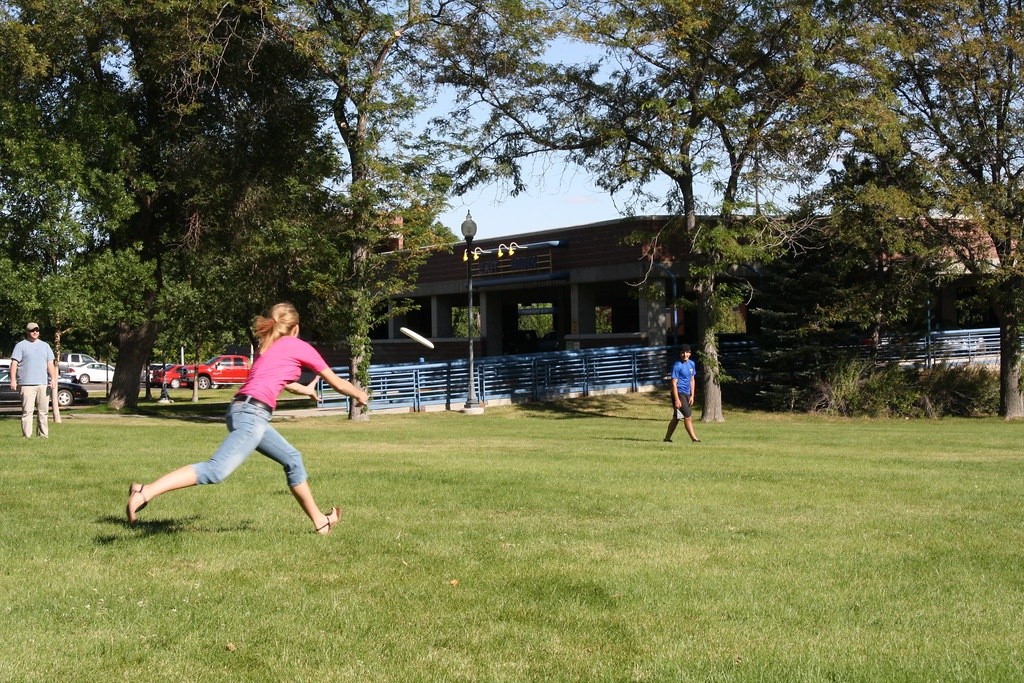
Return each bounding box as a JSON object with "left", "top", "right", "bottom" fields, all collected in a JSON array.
[{"left": 30, "top": 328, "right": 39, "bottom": 332}]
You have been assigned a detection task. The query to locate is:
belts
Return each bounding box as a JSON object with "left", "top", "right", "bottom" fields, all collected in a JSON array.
[{"left": 234, "top": 396, "right": 272, "bottom": 414}]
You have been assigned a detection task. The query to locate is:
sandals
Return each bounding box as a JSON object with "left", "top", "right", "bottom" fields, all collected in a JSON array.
[
  {"left": 316, "top": 508, "right": 341, "bottom": 535},
  {"left": 126, "top": 482, "right": 148, "bottom": 528}
]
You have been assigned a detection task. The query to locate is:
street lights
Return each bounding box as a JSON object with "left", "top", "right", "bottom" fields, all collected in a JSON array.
[{"left": 461, "top": 211, "right": 483, "bottom": 409}]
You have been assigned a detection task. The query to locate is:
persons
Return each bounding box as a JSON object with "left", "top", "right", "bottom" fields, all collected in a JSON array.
[
  {"left": 128, "top": 302, "right": 367, "bottom": 536},
  {"left": 10, "top": 322, "right": 57, "bottom": 441},
  {"left": 664, "top": 344, "right": 702, "bottom": 443}
]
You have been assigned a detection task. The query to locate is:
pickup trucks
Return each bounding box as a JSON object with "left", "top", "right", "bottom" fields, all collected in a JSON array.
[{"left": 179, "top": 355, "right": 251, "bottom": 390}]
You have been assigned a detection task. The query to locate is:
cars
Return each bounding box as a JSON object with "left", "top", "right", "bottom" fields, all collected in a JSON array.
[
  {"left": 934, "top": 327, "right": 988, "bottom": 358},
  {"left": 0, "top": 352, "right": 186, "bottom": 407}
]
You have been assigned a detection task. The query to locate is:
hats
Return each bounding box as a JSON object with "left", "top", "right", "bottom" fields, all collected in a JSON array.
[{"left": 26, "top": 322, "right": 40, "bottom": 331}]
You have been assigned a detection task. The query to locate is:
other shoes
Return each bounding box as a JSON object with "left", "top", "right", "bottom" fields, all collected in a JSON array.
[
  {"left": 664, "top": 439, "right": 673, "bottom": 442},
  {"left": 692, "top": 439, "right": 702, "bottom": 442}
]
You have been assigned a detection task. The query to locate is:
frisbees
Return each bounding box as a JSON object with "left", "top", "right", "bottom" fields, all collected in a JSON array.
[{"left": 399, "top": 326, "right": 434, "bottom": 349}]
